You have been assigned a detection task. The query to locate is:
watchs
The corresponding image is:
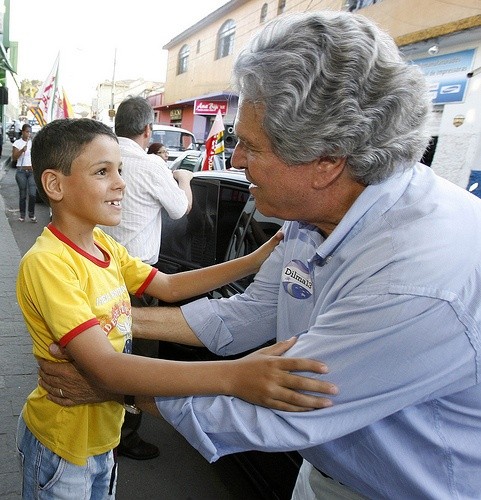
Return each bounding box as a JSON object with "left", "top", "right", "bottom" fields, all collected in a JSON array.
[{"left": 121, "top": 395, "right": 141, "bottom": 415}]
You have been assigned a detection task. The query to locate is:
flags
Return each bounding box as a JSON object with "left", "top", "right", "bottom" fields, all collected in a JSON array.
[
  {"left": 197, "top": 108, "right": 226, "bottom": 170},
  {"left": 28, "top": 58, "right": 76, "bottom": 128}
]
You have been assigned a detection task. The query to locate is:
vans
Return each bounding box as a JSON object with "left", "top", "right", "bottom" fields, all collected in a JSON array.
[{"left": 143, "top": 124, "right": 195, "bottom": 169}]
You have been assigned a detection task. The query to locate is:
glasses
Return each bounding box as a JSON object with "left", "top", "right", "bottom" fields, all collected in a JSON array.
[{"left": 157, "top": 150, "right": 169, "bottom": 155}]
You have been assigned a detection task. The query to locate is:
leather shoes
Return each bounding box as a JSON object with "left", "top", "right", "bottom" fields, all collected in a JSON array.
[{"left": 117, "top": 433, "right": 160, "bottom": 460}]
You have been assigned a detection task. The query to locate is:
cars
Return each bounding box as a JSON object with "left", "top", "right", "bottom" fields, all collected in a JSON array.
[
  {"left": 168, "top": 140, "right": 234, "bottom": 177},
  {"left": 153, "top": 170, "right": 303, "bottom": 500},
  {"left": 6, "top": 120, "right": 41, "bottom": 144}
]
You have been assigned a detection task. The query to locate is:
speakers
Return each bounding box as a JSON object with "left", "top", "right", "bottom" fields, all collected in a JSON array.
[{"left": 224, "top": 123, "right": 235, "bottom": 148}]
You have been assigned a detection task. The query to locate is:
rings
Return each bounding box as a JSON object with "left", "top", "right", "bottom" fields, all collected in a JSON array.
[{"left": 59, "top": 388, "right": 64, "bottom": 399}]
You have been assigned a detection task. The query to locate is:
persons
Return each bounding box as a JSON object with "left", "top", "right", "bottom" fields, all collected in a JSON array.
[
  {"left": 147, "top": 142, "right": 169, "bottom": 163},
  {"left": 12, "top": 124, "right": 39, "bottom": 223},
  {"left": 93, "top": 95, "right": 195, "bottom": 459},
  {"left": 15, "top": 117, "right": 340, "bottom": 500},
  {"left": 35, "top": 8, "right": 480, "bottom": 500}
]
